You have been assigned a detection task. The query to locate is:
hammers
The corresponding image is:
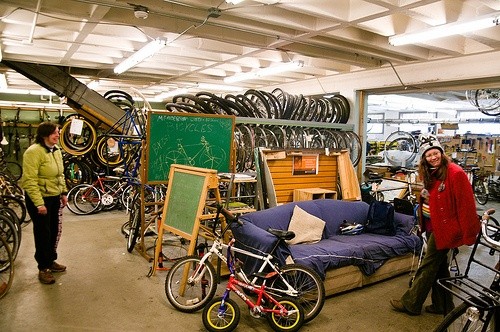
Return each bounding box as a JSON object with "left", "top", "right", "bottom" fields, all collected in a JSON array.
[{"left": 477, "top": 137, "right": 481, "bottom": 150}]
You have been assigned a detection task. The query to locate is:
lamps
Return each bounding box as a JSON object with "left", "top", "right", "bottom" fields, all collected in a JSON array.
[
  {"left": 114, "top": 36, "right": 167, "bottom": 74},
  {"left": 387, "top": 11, "right": 500, "bottom": 46}
]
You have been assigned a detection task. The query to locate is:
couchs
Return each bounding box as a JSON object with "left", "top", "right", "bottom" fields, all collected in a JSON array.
[{"left": 228, "top": 199, "right": 426, "bottom": 305}]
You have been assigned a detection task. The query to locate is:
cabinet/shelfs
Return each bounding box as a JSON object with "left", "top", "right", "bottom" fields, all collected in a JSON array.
[{"left": 293, "top": 188, "right": 337, "bottom": 201}]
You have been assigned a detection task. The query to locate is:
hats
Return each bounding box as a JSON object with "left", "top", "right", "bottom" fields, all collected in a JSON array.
[{"left": 419, "top": 133, "right": 443, "bottom": 157}]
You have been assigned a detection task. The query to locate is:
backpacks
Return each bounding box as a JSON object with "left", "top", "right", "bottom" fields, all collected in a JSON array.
[{"left": 365, "top": 200, "right": 397, "bottom": 236}]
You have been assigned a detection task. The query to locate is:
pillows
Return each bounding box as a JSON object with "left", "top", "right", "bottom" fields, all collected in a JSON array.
[{"left": 285, "top": 204, "right": 326, "bottom": 244}]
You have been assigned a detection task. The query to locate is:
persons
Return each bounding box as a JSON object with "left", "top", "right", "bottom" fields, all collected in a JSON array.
[
  {"left": 21, "top": 121, "right": 68, "bottom": 285},
  {"left": 390, "top": 133, "right": 478, "bottom": 317}
]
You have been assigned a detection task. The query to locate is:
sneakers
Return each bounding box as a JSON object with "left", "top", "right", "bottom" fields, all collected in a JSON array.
[
  {"left": 51, "top": 261, "right": 66, "bottom": 272},
  {"left": 39, "top": 269, "right": 55, "bottom": 284}
]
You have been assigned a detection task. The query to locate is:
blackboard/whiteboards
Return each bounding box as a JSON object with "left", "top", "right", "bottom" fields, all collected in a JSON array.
[
  {"left": 144, "top": 110, "right": 236, "bottom": 185},
  {"left": 161, "top": 163, "right": 217, "bottom": 240}
]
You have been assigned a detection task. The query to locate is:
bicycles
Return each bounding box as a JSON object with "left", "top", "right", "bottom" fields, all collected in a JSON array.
[
  {"left": 49, "top": 88, "right": 500, "bottom": 332},
  {"left": 0, "top": 103, "right": 31, "bottom": 301},
  {"left": 164, "top": 198, "right": 326, "bottom": 324},
  {"left": 201, "top": 256, "right": 305, "bottom": 332}
]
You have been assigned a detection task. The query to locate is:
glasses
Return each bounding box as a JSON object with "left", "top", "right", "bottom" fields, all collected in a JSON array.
[{"left": 424, "top": 150, "right": 441, "bottom": 159}]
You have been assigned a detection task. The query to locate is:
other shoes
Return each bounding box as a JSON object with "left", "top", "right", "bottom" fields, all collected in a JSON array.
[
  {"left": 425, "top": 304, "right": 455, "bottom": 314},
  {"left": 390, "top": 299, "right": 421, "bottom": 316}
]
